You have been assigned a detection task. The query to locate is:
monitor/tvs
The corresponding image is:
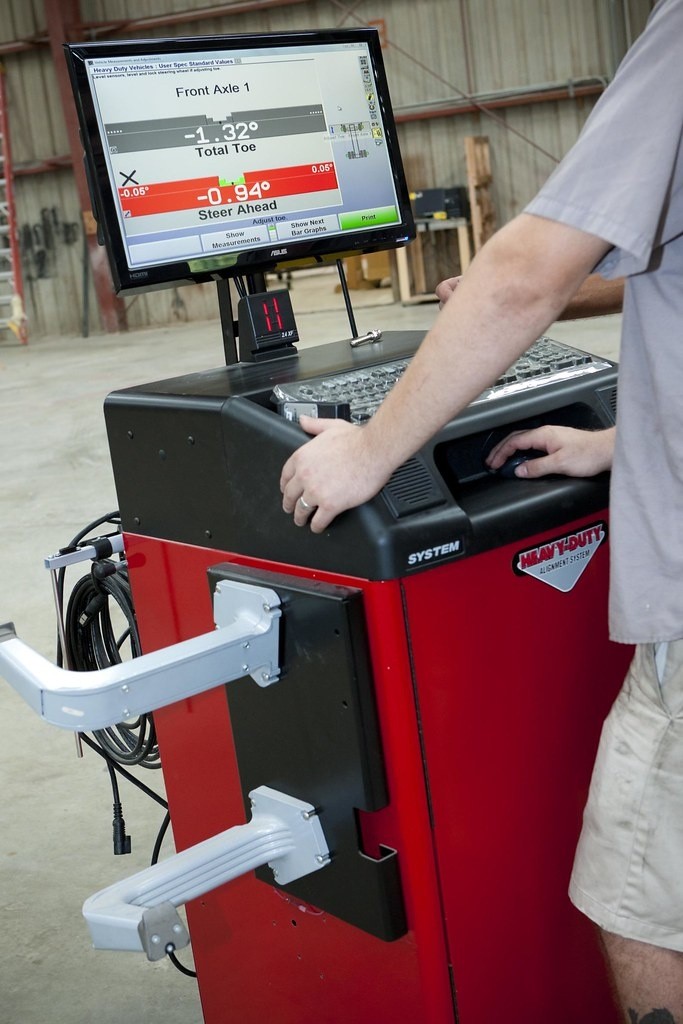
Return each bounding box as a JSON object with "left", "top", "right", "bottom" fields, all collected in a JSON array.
[{"left": 64, "top": 25, "right": 417, "bottom": 296}]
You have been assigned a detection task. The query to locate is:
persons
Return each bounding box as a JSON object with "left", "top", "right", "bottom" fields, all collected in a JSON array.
[{"left": 279, "top": 0, "right": 683, "bottom": 1024}]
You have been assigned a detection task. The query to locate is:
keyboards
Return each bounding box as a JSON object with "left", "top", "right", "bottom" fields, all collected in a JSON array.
[{"left": 274, "top": 334, "right": 612, "bottom": 418}]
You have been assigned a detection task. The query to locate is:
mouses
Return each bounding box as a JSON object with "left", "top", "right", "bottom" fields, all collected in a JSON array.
[{"left": 493, "top": 447, "right": 549, "bottom": 479}]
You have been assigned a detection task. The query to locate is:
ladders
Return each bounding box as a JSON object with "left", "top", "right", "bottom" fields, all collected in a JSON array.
[{"left": 0, "top": 67, "right": 27, "bottom": 346}]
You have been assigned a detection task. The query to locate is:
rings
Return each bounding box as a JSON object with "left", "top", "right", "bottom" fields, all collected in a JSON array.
[{"left": 299, "top": 497, "right": 316, "bottom": 511}]
